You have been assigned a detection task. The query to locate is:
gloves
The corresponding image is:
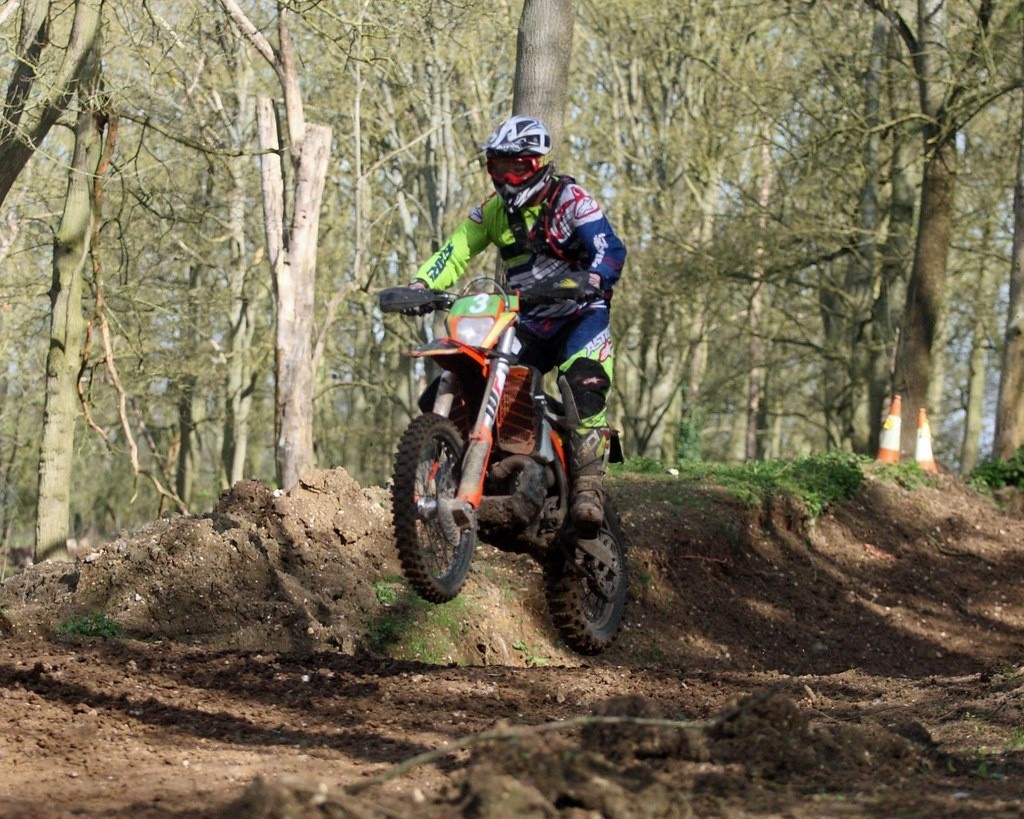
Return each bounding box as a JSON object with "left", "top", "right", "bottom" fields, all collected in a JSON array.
[
  {"left": 399, "top": 278, "right": 434, "bottom": 317},
  {"left": 576, "top": 273, "right": 601, "bottom": 310}
]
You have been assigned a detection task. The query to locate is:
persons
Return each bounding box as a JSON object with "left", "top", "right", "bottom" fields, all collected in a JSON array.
[{"left": 401, "top": 115, "right": 627, "bottom": 539}]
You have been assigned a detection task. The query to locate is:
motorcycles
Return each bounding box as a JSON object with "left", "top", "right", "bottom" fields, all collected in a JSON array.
[{"left": 380, "top": 271, "right": 631, "bottom": 656}]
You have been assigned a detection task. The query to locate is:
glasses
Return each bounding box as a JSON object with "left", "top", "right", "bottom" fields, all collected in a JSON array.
[{"left": 486, "top": 157, "right": 541, "bottom": 184}]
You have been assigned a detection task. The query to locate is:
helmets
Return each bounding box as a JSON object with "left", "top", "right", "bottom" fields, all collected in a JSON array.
[{"left": 480, "top": 114, "right": 552, "bottom": 215}]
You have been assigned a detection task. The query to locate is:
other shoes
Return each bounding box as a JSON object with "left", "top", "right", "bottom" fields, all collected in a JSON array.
[{"left": 573, "top": 461, "right": 605, "bottom": 521}]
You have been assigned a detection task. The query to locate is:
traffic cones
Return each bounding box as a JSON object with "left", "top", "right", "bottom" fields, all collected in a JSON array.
[
  {"left": 914, "top": 407, "right": 937, "bottom": 474},
  {"left": 876, "top": 394, "right": 902, "bottom": 466}
]
